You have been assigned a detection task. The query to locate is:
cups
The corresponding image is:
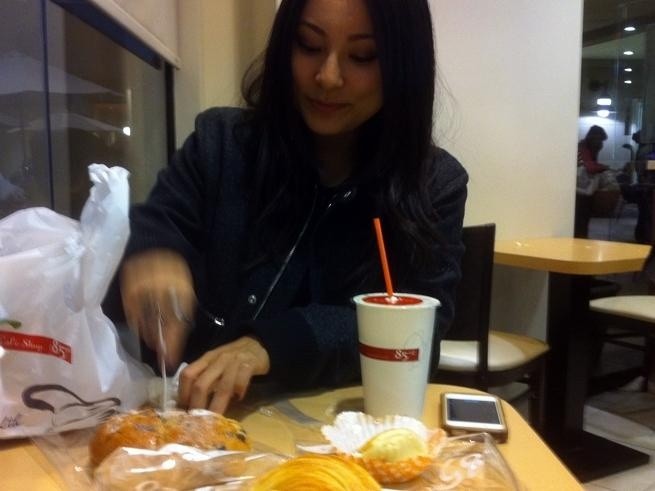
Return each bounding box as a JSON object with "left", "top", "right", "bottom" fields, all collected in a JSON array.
[{"left": 354, "top": 294, "right": 438, "bottom": 420}]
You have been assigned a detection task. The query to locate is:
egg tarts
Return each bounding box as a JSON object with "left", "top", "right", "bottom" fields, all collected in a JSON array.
[{"left": 361, "top": 427, "right": 426, "bottom": 468}]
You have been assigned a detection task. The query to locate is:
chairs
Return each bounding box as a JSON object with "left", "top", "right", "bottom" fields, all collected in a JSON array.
[
  {"left": 587, "top": 293, "right": 655, "bottom": 399},
  {"left": 617, "top": 172, "right": 638, "bottom": 217},
  {"left": 430, "top": 215, "right": 554, "bottom": 439}
]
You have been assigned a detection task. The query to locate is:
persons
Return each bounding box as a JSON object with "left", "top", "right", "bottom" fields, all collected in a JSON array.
[
  {"left": 574, "top": 125, "right": 611, "bottom": 238},
  {"left": 116, "top": 0, "right": 469, "bottom": 416}
]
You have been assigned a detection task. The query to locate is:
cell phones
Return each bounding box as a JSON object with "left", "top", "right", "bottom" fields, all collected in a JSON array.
[{"left": 440, "top": 392, "right": 508, "bottom": 440}]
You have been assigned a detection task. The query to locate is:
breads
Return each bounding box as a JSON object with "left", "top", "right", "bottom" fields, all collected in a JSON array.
[
  {"left": 248, "top": 454, "right": 379, "bottom": 491},
  {"left": 91, "top": 405, "right": 248, "bottom": 485}
]
